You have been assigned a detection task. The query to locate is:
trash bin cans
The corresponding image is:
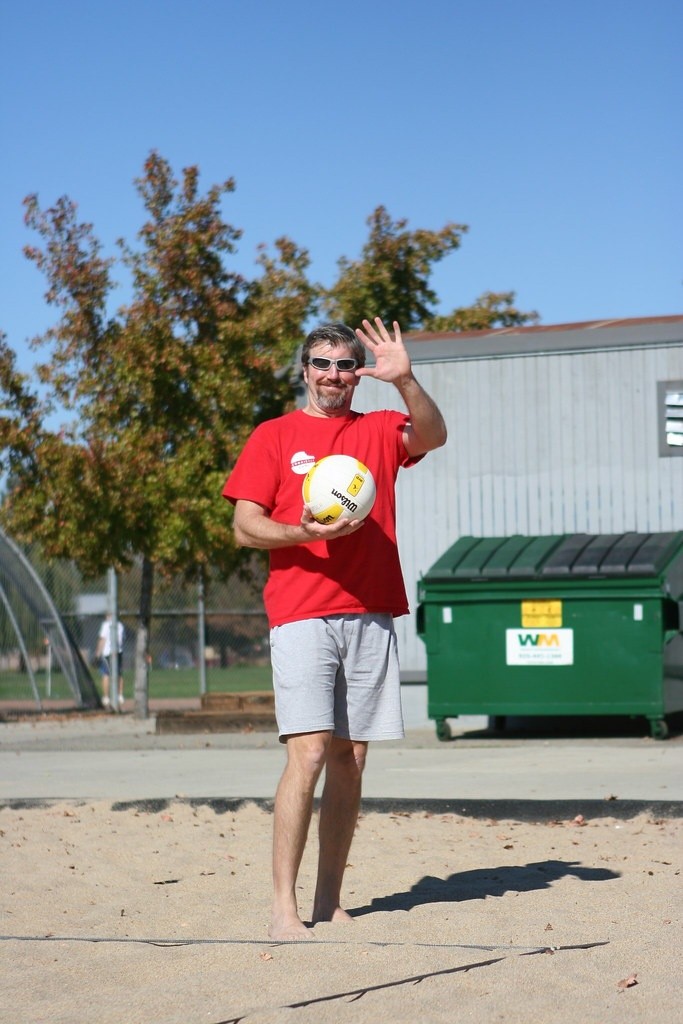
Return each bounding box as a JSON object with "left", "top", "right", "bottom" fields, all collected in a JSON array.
[{"left": 414, "top": 530, "right": 682, "bottom": 740}]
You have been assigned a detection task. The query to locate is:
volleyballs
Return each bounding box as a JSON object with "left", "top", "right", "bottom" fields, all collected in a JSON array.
[{"left": 301, "top": 452, "right": 378, "bottom": 530}]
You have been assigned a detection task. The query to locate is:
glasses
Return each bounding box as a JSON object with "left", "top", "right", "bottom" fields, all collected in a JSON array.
[{"left": 306, "top": 356, "right": 361, "bottom": 372}]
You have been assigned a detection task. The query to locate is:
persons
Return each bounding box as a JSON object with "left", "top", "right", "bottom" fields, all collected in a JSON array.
[
  {"left": 95, "top": 609, "right": 125, "bottom": 710},
  {"left": 220, "top": 317, "right": 447, "bottom": 943}
]
